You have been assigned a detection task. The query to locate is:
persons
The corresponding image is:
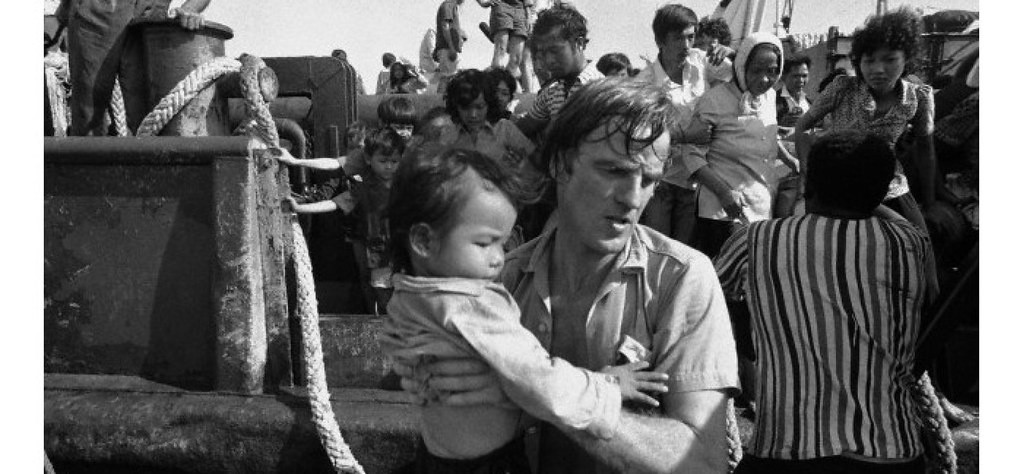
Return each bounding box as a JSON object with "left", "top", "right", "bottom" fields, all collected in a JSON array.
[
  {"left": 56, "top": 0, "right": 213, "bottom": 138},
  {"left": 270, "top": 0, "right": 979, "bottom": 427},
  {"left": 392, "top": 76, "right": 737, "bottom": 474},
  {"left": 714, "top": 128, "right": 932, "bottom": 474},
  {"left": 380, "top": 141, "right": 671, "bottom": 474}
]
